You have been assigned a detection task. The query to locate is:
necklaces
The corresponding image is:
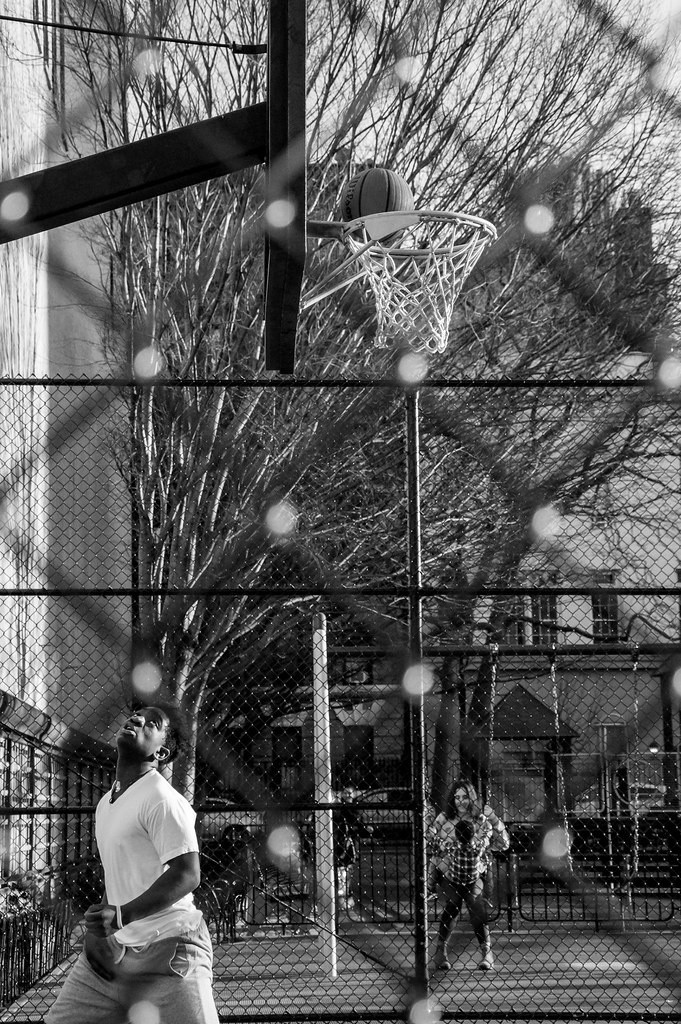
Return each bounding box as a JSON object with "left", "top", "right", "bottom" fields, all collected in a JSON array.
[{"left": 109, "top": 775, "right": 141, "bottom": 803}]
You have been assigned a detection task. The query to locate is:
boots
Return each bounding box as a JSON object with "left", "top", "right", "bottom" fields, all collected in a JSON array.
[
  {"left": 433, "top": 941, "right": 452, "bottom": 970},
  {"left": 477, "top": 943, "right": 494, "bottom": 970}
]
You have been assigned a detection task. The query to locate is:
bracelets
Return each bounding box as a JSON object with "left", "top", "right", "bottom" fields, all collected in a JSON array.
[{"left": 115, "top": 905, "right": 125, "bottom": 930}]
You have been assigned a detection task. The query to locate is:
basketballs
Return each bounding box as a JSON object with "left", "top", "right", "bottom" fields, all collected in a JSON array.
[{"left": 341, "top": 167, "right": 415, "bottom": 243}]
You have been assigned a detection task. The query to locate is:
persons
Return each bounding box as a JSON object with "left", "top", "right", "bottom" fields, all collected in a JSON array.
[
  {"left": 41, "top": 704, "right": 223, "bottom": 1024},
  {"left": 336, "top": 786, "right": 366, "bottom": 895},
  {"left": 424, "top": 779, "right": 511, "bottom": 971},
  {"left": 427, "top": 814, "right": 496, "bottom": 908}
]
